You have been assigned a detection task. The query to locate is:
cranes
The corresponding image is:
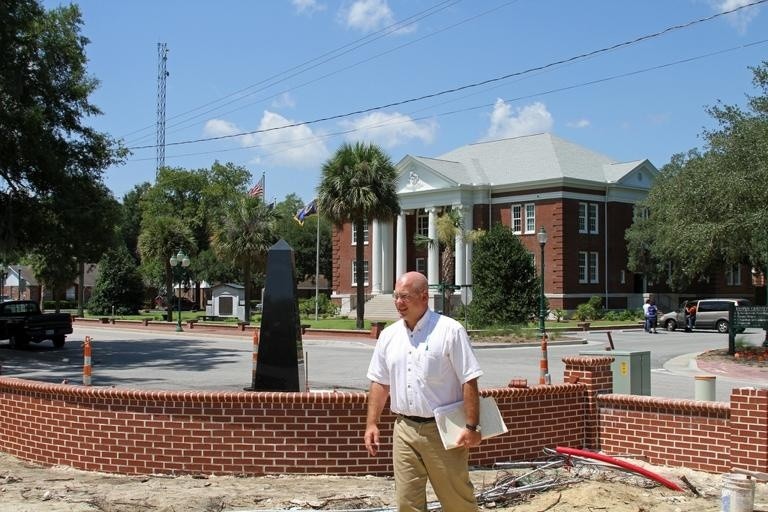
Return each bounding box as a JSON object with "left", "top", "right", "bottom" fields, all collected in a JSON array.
[{"left": 157, "top": 43, "right": 167, "bottom": 183}]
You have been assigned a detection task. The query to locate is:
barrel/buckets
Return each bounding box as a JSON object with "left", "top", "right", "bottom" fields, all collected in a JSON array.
[
  {"left": 720, "top": 472, "right": 756, "bottom": 512},
  {"left": 693, "top": 375, "right": 717, "bottom": 400}
]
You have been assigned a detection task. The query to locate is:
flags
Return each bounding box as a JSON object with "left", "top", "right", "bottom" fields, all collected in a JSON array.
[
  {"left": 247, "top": 177, "right": 264, "bottom": 200},
  {"left": 293, "top": 197, "right": 318, "bottom": 227},
  {"left": 266, "top": 201, "right": 275, "bottom": 211}
]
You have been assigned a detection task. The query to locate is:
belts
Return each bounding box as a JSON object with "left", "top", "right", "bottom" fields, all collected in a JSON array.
[{"left": 399, "top": 413, "right": 435, "bottom": 423}]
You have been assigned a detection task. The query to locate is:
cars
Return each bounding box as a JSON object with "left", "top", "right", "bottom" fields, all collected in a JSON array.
[{"left": 172, "top": 297, "right": 197, "bottom": 311}]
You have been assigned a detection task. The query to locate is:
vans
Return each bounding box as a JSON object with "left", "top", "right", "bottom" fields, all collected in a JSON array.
[{"left": 658, "top": 299, "right": 749, "bottom": 333}]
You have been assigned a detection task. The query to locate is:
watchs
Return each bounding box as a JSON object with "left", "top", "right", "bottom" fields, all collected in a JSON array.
[{"left": 465, "top": 424, "right": 482, "bottom": 432}]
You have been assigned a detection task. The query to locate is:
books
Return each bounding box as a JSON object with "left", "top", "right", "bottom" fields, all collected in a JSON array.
[{"left": 433, "top": 396, "right": 508, "bottom": 451}]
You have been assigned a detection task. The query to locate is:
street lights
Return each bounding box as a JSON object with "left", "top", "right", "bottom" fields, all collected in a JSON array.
[
  {"left": 170, "top": 248, "right": 191, "bottom": 332},
  {"left": 536, "top": 226, "right": 549, "bottom": 338}
]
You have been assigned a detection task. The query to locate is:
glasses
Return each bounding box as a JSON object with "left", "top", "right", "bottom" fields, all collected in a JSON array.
[{"left": 391, "top": 292, "right": 420, "bottom": 301}]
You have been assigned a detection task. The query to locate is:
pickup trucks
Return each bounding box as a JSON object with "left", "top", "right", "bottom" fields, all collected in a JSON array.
[{"left": 0, "top": 301, "right": 73, "bottom": 351}]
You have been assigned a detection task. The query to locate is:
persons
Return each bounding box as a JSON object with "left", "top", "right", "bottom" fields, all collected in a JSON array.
[
  {"left": 362, "top": 272, "right": 484, "bottom": 512},
  {"left": 686, "top": 304, "right": 696, "bottom": 329},
  {"left": 647, "top": 300, "right": 658, "bottom": 334},
  {"left": 642, "top": 300, "right": 650, "bottom": 332}
]
